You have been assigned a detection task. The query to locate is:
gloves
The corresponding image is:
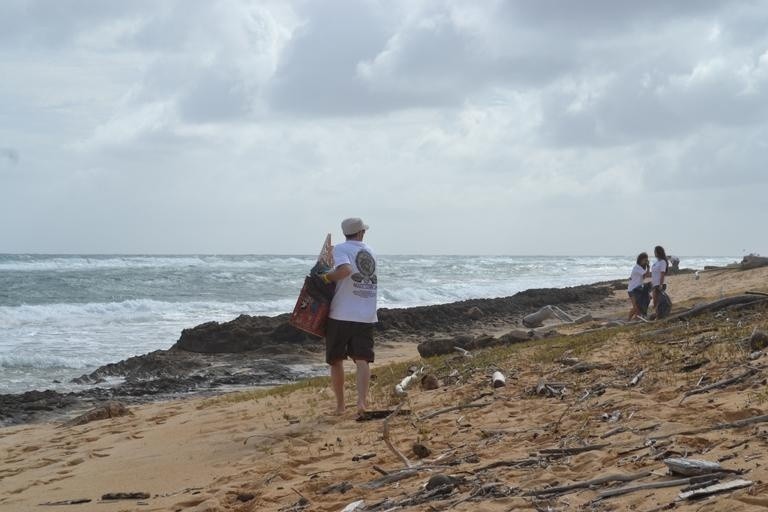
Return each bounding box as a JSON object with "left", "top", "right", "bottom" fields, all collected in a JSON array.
[{"left": 319, "top": 274, "right": 333, "bottom": 286}]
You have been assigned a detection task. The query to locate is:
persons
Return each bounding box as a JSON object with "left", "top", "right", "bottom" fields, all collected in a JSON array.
[
  {"left": 319, "top": 217, "right": 378, "bottom": 415},
  {"left": 628, "top": 246, "right": 668, "bottom": 321},
  {"left": 666, "top": 255, "right": 680, "bottom": 272}
]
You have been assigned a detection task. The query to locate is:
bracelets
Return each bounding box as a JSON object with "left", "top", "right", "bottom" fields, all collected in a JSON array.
[{"left": 323, "top": 274, "right": 331, "bottom": 285}]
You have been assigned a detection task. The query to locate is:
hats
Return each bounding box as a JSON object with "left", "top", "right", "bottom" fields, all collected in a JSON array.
[{"left": 341, "top": 217, "right": 369, "bottom": 235}]
